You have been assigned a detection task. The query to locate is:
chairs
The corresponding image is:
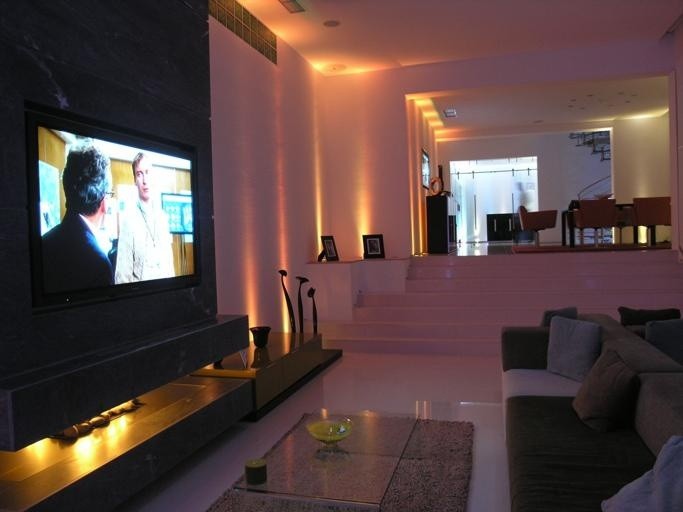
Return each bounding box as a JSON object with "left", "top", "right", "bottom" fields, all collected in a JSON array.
[{"left": 518, "top": 196, "right": 672, "bottom": 249}]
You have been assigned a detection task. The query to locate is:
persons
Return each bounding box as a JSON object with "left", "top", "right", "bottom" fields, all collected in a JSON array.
[
  {"left": 512, "top": 178, "right": 535, "bottom": 244},
  {"left": 39, "top": 143, "right": 112, "bottom": 291},
  {"left": 111, "top": 149, "right": 177, "bottom": 288}
]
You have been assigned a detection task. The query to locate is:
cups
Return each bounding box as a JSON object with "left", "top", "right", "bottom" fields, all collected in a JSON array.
[
  {"left": 245, "top": 459, "right": 267, "bottom": 487},
  {"left": 249, "top": 325, "right": 271, "bottom": 347}
]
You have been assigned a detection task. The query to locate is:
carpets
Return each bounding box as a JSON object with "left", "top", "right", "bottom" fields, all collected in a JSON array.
[{"left": 204, "top": 410, "right": 475, "bottom": 511}]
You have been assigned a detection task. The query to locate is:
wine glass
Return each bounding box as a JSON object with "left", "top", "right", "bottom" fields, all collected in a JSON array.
[{"left": 304, "top": 415, "right": 353, "bottom": 452}]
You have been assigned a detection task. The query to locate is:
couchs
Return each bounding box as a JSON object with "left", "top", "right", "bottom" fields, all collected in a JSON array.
[{"left": 501, "top": 313, "right": 683, "bottom": 511}]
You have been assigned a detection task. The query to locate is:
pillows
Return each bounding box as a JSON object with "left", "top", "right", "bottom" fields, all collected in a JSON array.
[
  {"left": 618, "top": 305, "right": 682, "bottom": 370},
  {"left": 599, "top": 435, "right": 683, "bottom": 511},
  {"left": 545, "top": 315, "right": 643, "bottom": 431}
]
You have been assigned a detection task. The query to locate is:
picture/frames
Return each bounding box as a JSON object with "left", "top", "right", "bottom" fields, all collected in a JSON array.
[
  {"left": 321, "top": 235, "right": 340, "bottom": 261},
  {"left": 362, "top": 234, "right": 385, "bottom": 258}
]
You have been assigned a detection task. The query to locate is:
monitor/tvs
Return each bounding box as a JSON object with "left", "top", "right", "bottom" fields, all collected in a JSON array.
[{"left": 29, "top": 109, "right": 202, "bottom": 313}]
